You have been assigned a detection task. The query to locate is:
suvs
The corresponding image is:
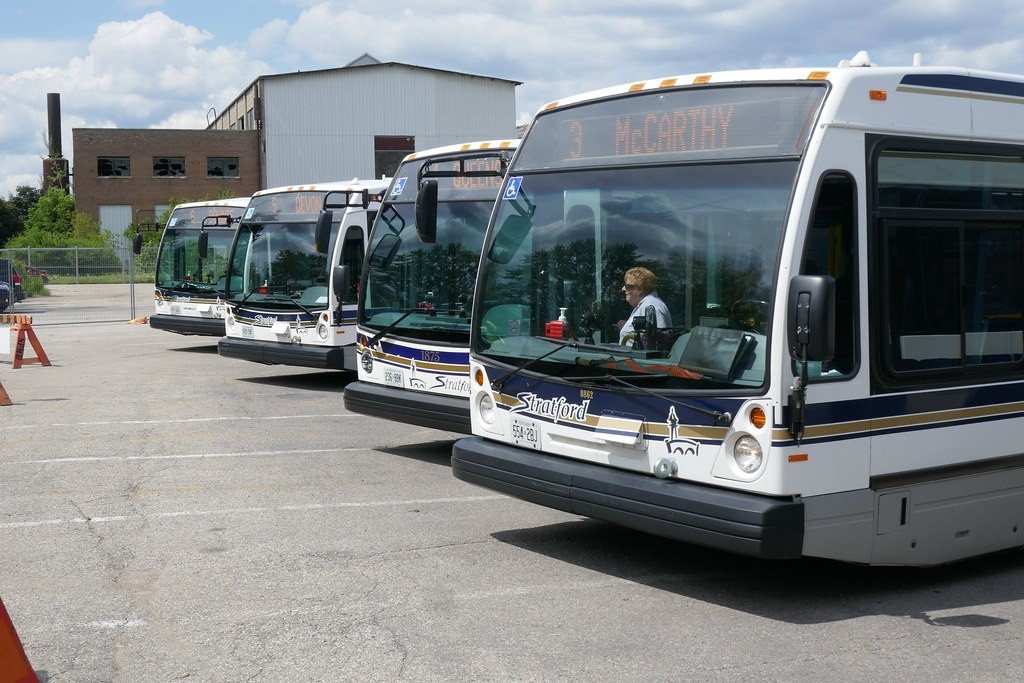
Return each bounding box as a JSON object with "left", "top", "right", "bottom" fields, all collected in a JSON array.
[
  {"left": 0, "top": 282, "right": 15, "bottom": 314},
  {"left": 0, "top": 258, "right": 27, "bottom": 316}
]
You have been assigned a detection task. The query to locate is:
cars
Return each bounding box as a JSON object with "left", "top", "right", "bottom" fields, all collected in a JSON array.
[{"left": 25, "top": 265, "right": 49, "bottom": 285}]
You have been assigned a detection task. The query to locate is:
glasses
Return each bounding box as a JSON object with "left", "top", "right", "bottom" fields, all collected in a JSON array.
[{"left": 624, "top": 283, "right": 645, "bottom": 290}]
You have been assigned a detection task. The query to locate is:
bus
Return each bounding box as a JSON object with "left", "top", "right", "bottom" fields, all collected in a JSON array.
[
  {"left": 198, "top": 179, "right": 396, "bottom": 373},
  {"left": 132, "top": 198, "right": 251, "bottom": 337},
  {"left": 415, "top": 50, "right": 1024, "bottom": 566},
  {"left": 314, "top": 139, "right": 605, "bottom": 434}
]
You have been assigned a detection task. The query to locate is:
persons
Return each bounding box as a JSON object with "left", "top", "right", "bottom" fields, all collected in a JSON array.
[{"left": 611, "top": 267, "right": 673, "bottom": 349}]
